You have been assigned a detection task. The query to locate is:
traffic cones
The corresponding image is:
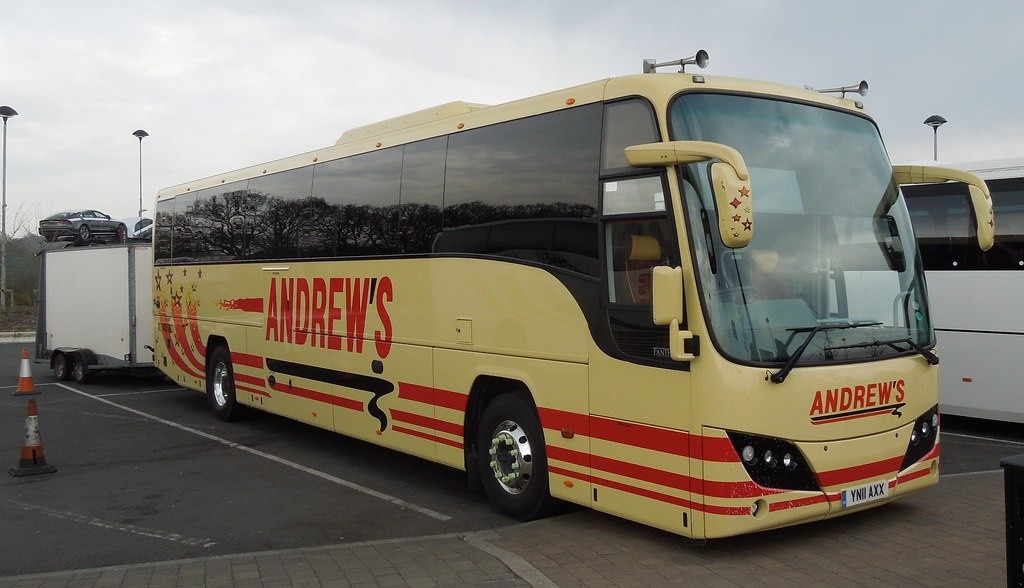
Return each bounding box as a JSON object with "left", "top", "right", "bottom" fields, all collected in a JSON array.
[
  {"left": 8, "top": 346, "right": 47, "bottom": 399},
  {"left": 6, "top": 399, "right": 58, "bottom": 477}
]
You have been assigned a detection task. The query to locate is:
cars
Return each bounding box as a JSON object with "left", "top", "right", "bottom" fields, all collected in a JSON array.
[{"left": 37, "top": 208, "right": 130, "bottom": 244}]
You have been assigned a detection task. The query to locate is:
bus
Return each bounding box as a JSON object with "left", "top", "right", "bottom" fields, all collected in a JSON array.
[
  {"left": 143, "top": 53, "right": 997, "bottom": 546},
  {"left": 641, "top": 158, "right": 1024, "bottom": 432}
]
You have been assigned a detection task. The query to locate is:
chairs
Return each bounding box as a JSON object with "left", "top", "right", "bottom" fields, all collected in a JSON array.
[{"left": 615, "top": 232, "right": 664, "bottom": 307}]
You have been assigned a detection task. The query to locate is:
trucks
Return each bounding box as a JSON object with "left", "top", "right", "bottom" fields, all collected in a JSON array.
[{"left": 32, "top": 241, "right": 169, "bottom": 384}]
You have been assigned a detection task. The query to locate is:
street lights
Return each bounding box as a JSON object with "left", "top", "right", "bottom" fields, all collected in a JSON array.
[
  {"left": 132, "top": 129, "right": 152, "bottom": 224},
  {"left": 0, "top": 106, "right": 19, "bottom": 310},
  {"left": 922, "top": 113, "right": 947, "bottom": 160}
]
